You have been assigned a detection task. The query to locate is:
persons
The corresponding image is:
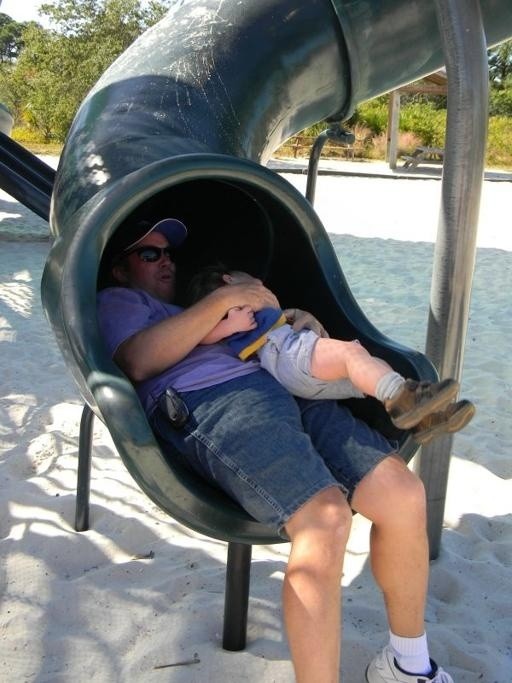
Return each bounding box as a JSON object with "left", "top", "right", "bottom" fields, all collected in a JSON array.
[
  {"left": 186, "top": 258, "right": 476, "bottom": 444},
  {"left": 95, "top": 217, "right": 455, "bottom": 683}
]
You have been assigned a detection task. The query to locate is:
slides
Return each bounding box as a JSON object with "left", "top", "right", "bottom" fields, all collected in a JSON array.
[
  {"left": 40, "top": 0, "right": 512, "bottom": 546},
  {"left": 0, "top": 132, "right": 57, "bottom": 222}
]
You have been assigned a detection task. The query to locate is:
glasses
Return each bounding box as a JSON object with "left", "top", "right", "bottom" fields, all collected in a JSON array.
[{"left": 120, "top": 245, "right": 178, "bottom": 262}]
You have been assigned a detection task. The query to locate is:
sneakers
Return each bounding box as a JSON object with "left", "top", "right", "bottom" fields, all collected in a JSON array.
[
  {"left": 365, "top": 644, "right": 455, "bottom": 683},
  {"left": 384, "top": 378, "right": 475, "bottom": 445}
]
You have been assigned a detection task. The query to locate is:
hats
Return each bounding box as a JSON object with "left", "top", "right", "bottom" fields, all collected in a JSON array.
[{"left": 99, "top": 218, "right": 188, "bottom": 270}]
